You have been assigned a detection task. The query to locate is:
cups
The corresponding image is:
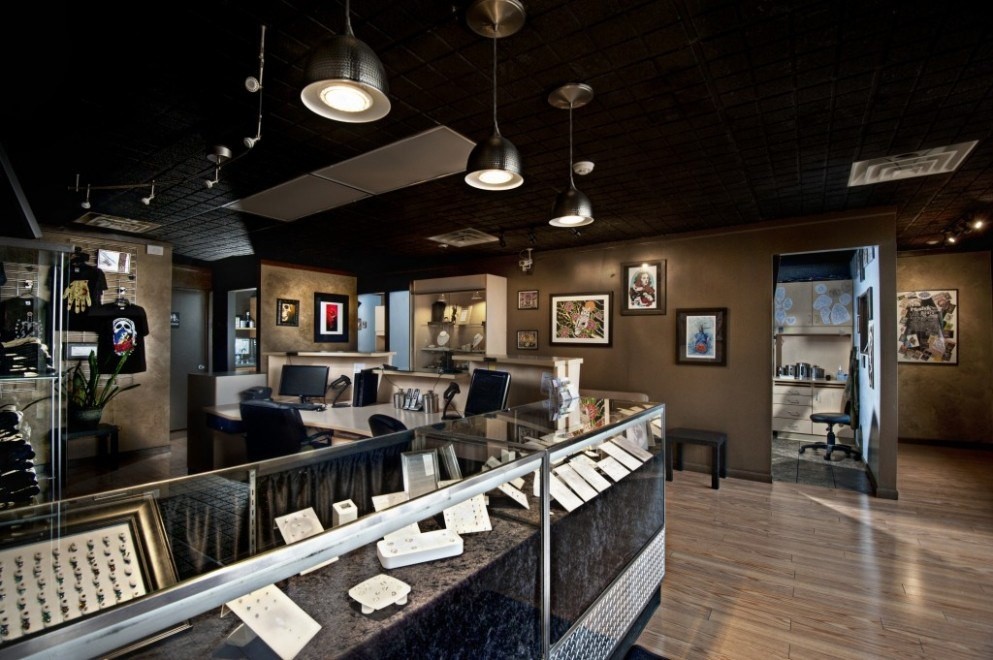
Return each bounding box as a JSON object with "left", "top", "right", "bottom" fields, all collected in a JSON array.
[
  {"left": 422, "top": 390, "right": 438, "bottom": 413},
  {"left": 777, "top": 363, "right": 825, "bottom": 379},
  {"left": 393, "top": 389, "right": 407, "bottom": 409}
]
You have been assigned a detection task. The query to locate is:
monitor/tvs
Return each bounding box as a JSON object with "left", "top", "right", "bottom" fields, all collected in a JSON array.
[{"left": 277, "top": 363, "right": 330, "bottom": 403}]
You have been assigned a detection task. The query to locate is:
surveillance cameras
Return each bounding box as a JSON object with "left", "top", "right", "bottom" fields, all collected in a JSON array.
[{"left": 520, "top": 258, "right": 535, "bottom": 273}]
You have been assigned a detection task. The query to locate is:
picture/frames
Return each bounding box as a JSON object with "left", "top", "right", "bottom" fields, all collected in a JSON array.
[
  {"left": 2, "top": 491, "right": 196, "bottom": 659},
  {"left": 275, "top": 298, "right": 300, "bottom": 327},
  {"left": 455, "top": 305, "right": 472, "bottom": 325},
  {"left": 897, "top": 289, "right": 959, "bottom": 366},
  {"left": 313, "top": 291, "right": 352, "bottom": 344},
  {"left": 546, "top": 292, "right": 612, "bottom": 349},
  {"left": 619, "top": 260, "right": 667, "bottom": 317},
  {"left": 516, "top": 328, "right": 539, "bottom": 351},
  {"left": 674, "top": 306, "right": 727, "bottom": 367},
  {"left": 515, "top": 290, "right": 540, "bottom": 311}
]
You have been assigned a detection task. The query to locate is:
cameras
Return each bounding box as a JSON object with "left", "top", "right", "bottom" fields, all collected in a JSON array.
[{"left": 463, "top": 368, "right": 511, "bottom": 416}]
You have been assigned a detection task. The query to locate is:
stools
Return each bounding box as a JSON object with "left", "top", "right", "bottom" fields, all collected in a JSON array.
[{"left": 665, "top": 427, "right": 729, "bottom": 491}]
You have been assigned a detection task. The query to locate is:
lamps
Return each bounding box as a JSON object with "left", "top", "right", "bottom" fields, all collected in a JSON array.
[
  {"left": 547, "top": 81, "right": 597, "bottom": 230},
  {"left": 299, "top": 0, "right": 392, "bottom": 125},
  {"left": 74, "top": 20, "right": 265, "bottom": 209},
  {"left": 463, "top": 0, "right": 530, "bottom": 191}
]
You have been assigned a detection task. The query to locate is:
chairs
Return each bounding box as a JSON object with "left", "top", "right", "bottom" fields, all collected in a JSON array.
[
  {"left": 241, "top": 398, "right": 335, "bottom": 462},
  {"left": 799, "top": 392, "right": 861, "bottom": 464}
]
